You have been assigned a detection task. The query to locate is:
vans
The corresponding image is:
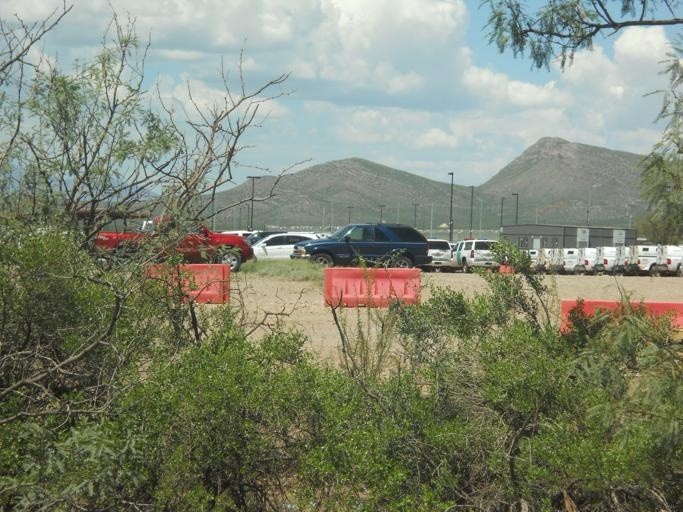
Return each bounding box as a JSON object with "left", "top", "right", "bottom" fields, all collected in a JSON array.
[{"left": 427, "top": 239, "right": 682, "bottom": 275}]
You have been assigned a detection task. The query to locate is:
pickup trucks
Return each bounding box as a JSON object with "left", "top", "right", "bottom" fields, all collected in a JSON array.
[{"left": 97, "top": 216, "right": 254, "bottom": 272}]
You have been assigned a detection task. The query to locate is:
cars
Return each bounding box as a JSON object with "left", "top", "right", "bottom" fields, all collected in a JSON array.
[
  {"left": 219, "top": 229, "right": 322, "bottom": 261},
  {"left": 291, "top": 224, "right": 433, "bottom": 268}
]
